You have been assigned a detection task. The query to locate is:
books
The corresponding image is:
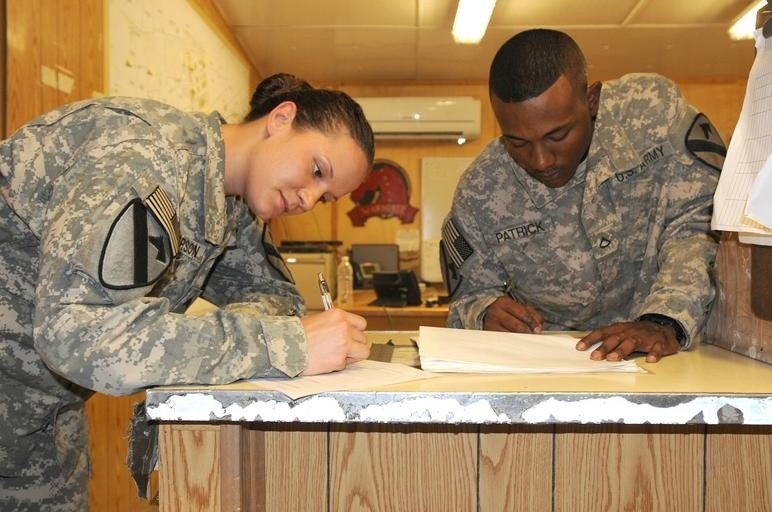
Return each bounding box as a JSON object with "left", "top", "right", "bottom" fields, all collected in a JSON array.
[
  {"left": 411, "top": 325, "right": 648, "bottom": 375},
  {"left": 260, "top": 352, "right": 432, "bottom": 402}
]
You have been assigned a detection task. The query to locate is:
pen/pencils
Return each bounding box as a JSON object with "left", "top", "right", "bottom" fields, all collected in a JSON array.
[
  {"left": 504, "top": 283, "right": 522, "bottom": 305},
  {"left": 317, "top": 272, "right": 333, "bottom": 310}
]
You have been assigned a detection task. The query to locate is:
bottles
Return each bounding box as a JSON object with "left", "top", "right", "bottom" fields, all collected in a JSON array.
[{"left": 336, "top": 256, "right": 354, "bottom": 308}]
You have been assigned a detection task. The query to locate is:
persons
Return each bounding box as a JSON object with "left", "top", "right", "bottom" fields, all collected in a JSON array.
[
  {"left": 1, "top": 71, "right": 377, "bottom": 512},
  {"left": 438, "top": 26, "right": 730, "bottom": 364}
]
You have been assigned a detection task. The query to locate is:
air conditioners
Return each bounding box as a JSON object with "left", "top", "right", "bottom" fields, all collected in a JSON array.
[{"left": 348, "top": 92, "right": 483, "bottom": 142}]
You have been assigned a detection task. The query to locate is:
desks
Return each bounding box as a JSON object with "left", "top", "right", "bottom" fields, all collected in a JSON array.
[
  {"left": 316, "top": 289, "right": 460, "bottom": 330},
  {"left": 143, "top": 330, "right": 772, "bottom": 510}
]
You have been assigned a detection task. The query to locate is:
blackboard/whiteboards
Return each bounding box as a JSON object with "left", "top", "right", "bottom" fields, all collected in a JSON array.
[{"left": 420, "top": 156, "right": 477, "bottom": 283}]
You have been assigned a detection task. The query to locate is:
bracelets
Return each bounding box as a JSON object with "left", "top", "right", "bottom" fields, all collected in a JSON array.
[{"left": 643, "top": 315, "right": 684, "bottom": 343}]
